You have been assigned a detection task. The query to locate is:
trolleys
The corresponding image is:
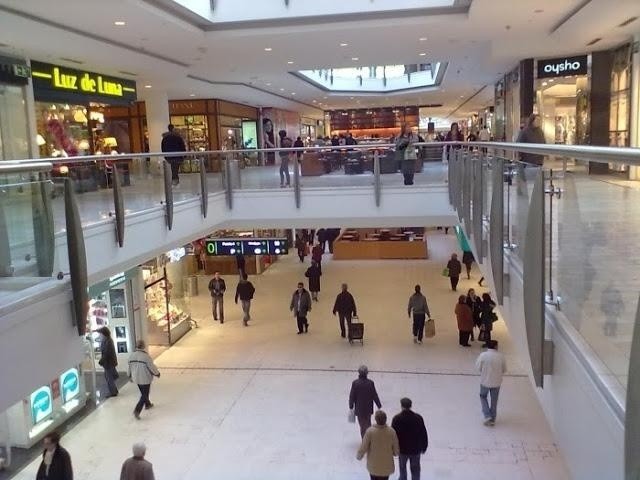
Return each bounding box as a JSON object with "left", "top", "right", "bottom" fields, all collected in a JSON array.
[{"left": 348, "top": 315, "right": 364, "bottom": 345}]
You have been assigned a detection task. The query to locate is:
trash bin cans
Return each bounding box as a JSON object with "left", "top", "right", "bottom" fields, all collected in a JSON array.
[
  {"left": 183, "top": 275, "right": 198, "bottom": 298},
  {"left": 221, "top": 159, "right": 241, "bottom": 190}
]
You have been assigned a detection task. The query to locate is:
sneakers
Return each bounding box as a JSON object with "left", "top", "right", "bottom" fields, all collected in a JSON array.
[
  {"left": 144, "top": 402, "right": 155, "bottom": 410},
  {"left": 478, "top": 281, "right": 482, "bottom": 287},
  {"left": 413, "top": 335, "right": 422, "bottom": 345},
  {"left": 242, "top": 319, "right": 248, "bottom": 326},
  {"left": 133, "top": 410, "right": 141, "bottom": 420},
  {"left": 297, "top": 324, "right": 309, "bottom": 334},
  {"left": 458, "top": 337, "right": 474, "bottom": 347},
  {"left": 214, "top": 318, "right": 224, "bottom": 323},
  {"left": 478, "top": 337, "right": 488, "bottom": 348},
  {"left": 340, "top": 332, "right": 346, "bottom": 337},
  {"left": 483, "top": 416, "right": 496, "bottom": 426},
  {"left": 104, "top": 391, "right": 119, "bottom": 398}
]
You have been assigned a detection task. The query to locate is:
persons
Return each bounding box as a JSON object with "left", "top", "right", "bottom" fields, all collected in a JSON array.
[
  {"left": 393, "top": 398, "right": 429, "bottom": 480},
  {"left": 119, "top": 442, "right": 155, "bottom": 480},
  {"left": 35, "top": 432, "right": 74, "bottom": 479},
  {"left": 455, "top": 294, "right": 475, "bottom": 347},
  {"left": 356, "top": 411, "right": 401, "bottom": 480},
  {"left": 447, "top": 253, "right": 462, "bottom": 292},
  {"left": 475, "top": 340, "right": 507, "bottom": 425},
  {"left": 95, "top": 326, "right": 119, "bottom": 398},
  {"left": 290, "top": 282, "right": 312, "bottom": 334},
  {"left": 263, "top": 118, "right": 491, "bottom": 189},
  {"left": 125, "top": 340, "right": 160, "bottom": 420},
  {"left": 349, "top": 364, "right": 382, "bottom": 441},
  {"left": 208, "top": 271, "right": 226, "bottom": 323},
  {"left": 191, "top": 228, "right": 341, "bottom": 302},
  {"left": 235, "top": 273, "right": 255, "bottom": 326},
  {"left": 160, "top": 122, "right": 186, "bottom": 184},
  {"left": 462, "top": 251, "right": 475, "bottom": 279},
  {"left": 333, "top": 284, "right": 357, "bottom": 338},
  {"left": 478, "top": 293, "right": 497, "bottom": 341},
  {"left": 513, "top": 114, "right": 546, "bottom": 168},
  {"left": 408, "top": 285, "right": 430, "bottom": 345},
  {"left": 466, "top": 288, "right": 482, "bottom": 341}
]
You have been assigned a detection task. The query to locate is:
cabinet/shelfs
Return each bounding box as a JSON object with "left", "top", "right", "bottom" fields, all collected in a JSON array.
[{"left": 144, "top": 272, "right": 191, "bottom": 346}]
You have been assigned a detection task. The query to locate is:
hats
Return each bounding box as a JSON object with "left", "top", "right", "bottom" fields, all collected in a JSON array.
[{"left": 399, "top": 397, "right": 413, "bottom": 407}]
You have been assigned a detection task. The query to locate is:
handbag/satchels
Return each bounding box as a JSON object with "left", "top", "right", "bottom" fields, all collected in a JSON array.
[
  {"left": 348, "top": 409, "right": 356, "bottom": 423},
  {"left": 491, "top": 312, "right": 498, "bottom": 322},
  {"left": 113, "top": 368, "right": 119, "bottom": 380},
  {"left": 424, "top": 318, "right": 436, "bottom": 338},
  {"left": 440, "top": 267, "right": 450, "bottom": 277}
]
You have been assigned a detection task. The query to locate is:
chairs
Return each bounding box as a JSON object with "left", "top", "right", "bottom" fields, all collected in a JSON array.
[{"left": 298, "top": 131, "right": 425, "bottom": 179}]
